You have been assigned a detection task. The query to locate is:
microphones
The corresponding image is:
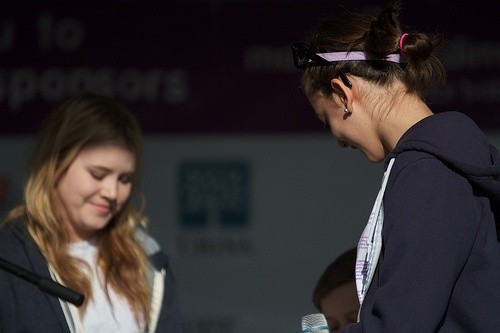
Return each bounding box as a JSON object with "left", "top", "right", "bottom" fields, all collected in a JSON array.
[{"left": 301, "top": 313, "right": 330, "bottom": 333}]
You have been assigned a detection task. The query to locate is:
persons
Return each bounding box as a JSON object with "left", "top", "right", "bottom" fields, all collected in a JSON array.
[
  {"left": 0, "top": 91, "right": 185, "bottom": 333},
  {"left": 313, "top": 247, "right": 360, "bottom": 333},
  {"left": 291, "top": 3, "right": 500, "bottom": 333}
]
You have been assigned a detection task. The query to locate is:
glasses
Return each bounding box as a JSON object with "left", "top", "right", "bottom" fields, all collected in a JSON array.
[{"left": 292, "top": 42, "right": 352, "bottom": 87}]
